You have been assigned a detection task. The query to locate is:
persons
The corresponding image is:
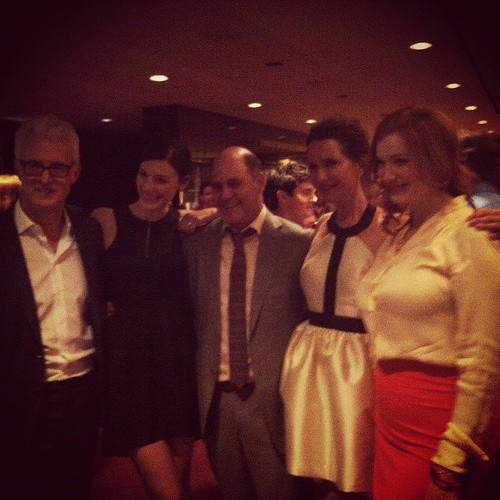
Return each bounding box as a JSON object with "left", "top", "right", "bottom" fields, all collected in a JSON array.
[
  {"left": 457, "top": 133, "right": 500, "bottom": 212},
  {"left": 194, "top": 180, "right": 215, "bottom": 211},
  {"left": 91, "top": 146, "right": 219, "bottom": 500},
  {"left": 349, "top": 105, "right": 500, "bottom": 500},
  {"left": 263, "top": 158, "right": 336, "bottom": 231},
  {"left": 0, "top": 118, "right": 112, "bottom": 499},
  {"left": 277, "top": 116, "right": 500, "bottom": 500},
  {"left": 184, "top": 146, "right": 314, "bottom": 500}
]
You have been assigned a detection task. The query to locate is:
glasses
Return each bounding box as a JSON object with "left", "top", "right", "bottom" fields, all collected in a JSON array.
[{"left": 17, "top": 157, "right": 74, "bottom": 178}]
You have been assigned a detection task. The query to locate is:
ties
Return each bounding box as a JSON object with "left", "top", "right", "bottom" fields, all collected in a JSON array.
[{"left": 225, "top": 226, "right": 256, "bottom": 385}]
